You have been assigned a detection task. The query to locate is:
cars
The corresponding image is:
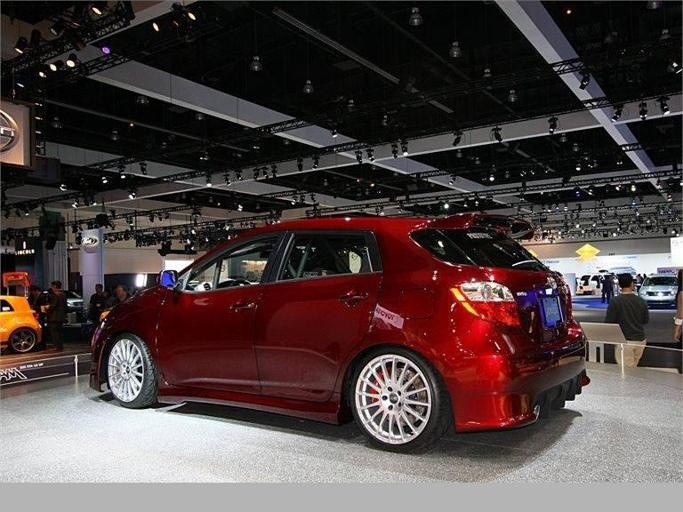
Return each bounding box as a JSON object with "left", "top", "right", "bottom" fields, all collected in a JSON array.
[
  {"left": 0, "top": 296, "right": 42, "bottom": 352},
  {"left": 36, "top": 289, "right": 84, "bottom": 313},
  {"left": 578, "top": 273, "right": 619, "bottom": 294},
  {"left": 637, "top": 272, "right": 678, "bottom": 308},
  {"left": 85, "top": 213, "right": 591, "bottom": 452}
]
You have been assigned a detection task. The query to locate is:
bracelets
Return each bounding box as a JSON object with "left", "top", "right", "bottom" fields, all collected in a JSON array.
[{"left": 673, "top": 317, "right": 682, "bottom": 326}]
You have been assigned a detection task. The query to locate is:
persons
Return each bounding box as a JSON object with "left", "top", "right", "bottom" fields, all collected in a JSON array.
[
  {"left": 605, "top": 274, "right": 649, "bottom": 368},
  {"left": 598, "top": 271, "right": 647, "bottom": 304},
  {"left": 670, "top": 269, "right": 682, "bottom": 349},
  {"left": 27, "top": 281, "right": 130, "bottom": 353}
]
